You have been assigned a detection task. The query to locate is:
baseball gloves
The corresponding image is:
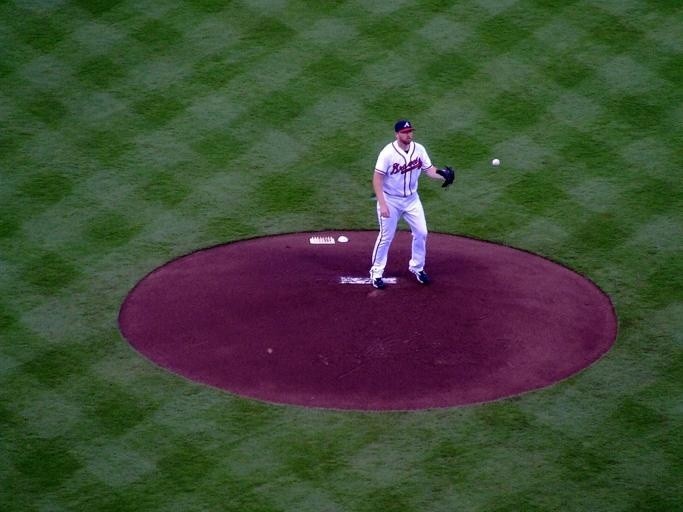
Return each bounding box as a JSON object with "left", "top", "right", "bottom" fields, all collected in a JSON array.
[{"left": 435, "top": 165, "right": 456, "bottom": 188}]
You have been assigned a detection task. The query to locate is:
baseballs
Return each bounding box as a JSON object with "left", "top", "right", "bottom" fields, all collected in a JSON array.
[{"left": 492, "top": 158, "right": 499, "bottom": 167}]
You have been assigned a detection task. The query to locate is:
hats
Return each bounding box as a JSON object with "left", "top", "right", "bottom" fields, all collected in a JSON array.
[{"left": 394, "top": 119, "right": 417, "bottom": 134}]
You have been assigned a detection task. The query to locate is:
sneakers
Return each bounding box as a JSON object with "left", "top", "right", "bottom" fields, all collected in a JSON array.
[
  {"left": 370, "top": 273, "right": 384, "bottom": 289},
  {"left": 408, "top": 266, "right": 432, "bottom": 285}
]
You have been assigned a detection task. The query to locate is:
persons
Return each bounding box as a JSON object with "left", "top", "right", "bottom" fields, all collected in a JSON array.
[{"left": 369, "top": 119, "right": 455, "bottom": 289}]
